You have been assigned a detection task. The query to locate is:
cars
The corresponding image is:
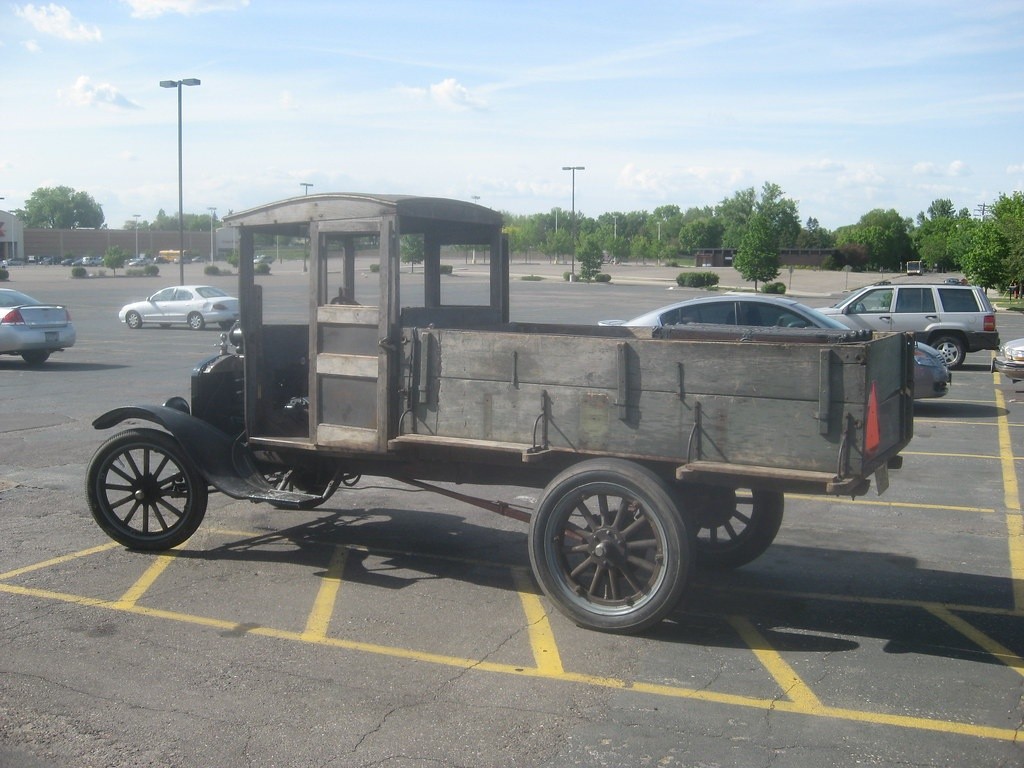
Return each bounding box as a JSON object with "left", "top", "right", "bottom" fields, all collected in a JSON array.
[
  {"left": 38, "top": 256, "right": 83, "bottom": 266},
  {"left": 126, "top": 258, "right": 145, "bottom": 266},
  {"left": 119, "top": 285, "right": 239, "bottom": 330},
  {"left": 253, "top": 255, "right": 275, "bottom": 264},
  {"left": 0, "top": 289, "right": 76, "bottom": 364},
  {"left": 598, "top": 295, "right": 952, "bottom": 402},
  {"left": 192, "top": 255, "right": 204, "bottom": 262},
  {"left": 991, "top": 337, "right": 1024, "bottom": 383},
  {"left": 944, "top": 278, "right": 960, "bottom": 284}
]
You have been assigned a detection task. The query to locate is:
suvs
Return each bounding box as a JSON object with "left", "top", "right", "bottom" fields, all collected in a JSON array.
[{"left": 778, "top": 283, "right": 1000, "bottom": 370}]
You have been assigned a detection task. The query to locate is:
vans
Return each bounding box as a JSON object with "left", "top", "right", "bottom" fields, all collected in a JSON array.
[{"left": 83, "top": 257, "right": 103, "bottom": 266}]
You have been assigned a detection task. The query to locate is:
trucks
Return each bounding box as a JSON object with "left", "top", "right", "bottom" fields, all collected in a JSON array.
[{"left": 84, "top": 192, "right": 913, "bottom": 635}]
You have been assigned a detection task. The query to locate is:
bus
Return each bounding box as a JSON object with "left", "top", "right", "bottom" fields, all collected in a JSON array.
[
  {"left": 159, "top": 249, "right": 190, "bottom": 263},
  {"left": 905, "top": 260, "right": 923, "bottom": 276}
]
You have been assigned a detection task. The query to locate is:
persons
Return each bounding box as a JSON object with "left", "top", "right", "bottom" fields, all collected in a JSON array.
[{"left": 864, "top": 294, "right": 892, "bottom": 311}]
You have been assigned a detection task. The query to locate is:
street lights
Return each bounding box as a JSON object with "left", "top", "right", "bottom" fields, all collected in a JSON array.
[
  {"left": 300, "top": 183, "right": 314, "bottom": 266},
  {"left": 472, "top": 195, "right": 480, "bottom": 259},
  {"left": 160, "top": 78, "right": 200, "bottom": 285},
  {"left": 562, "top": 167, "right": 585, "bottom": 274},
  {"left": 613, "top": 216, "right": 618, "bottom": 265}
]
